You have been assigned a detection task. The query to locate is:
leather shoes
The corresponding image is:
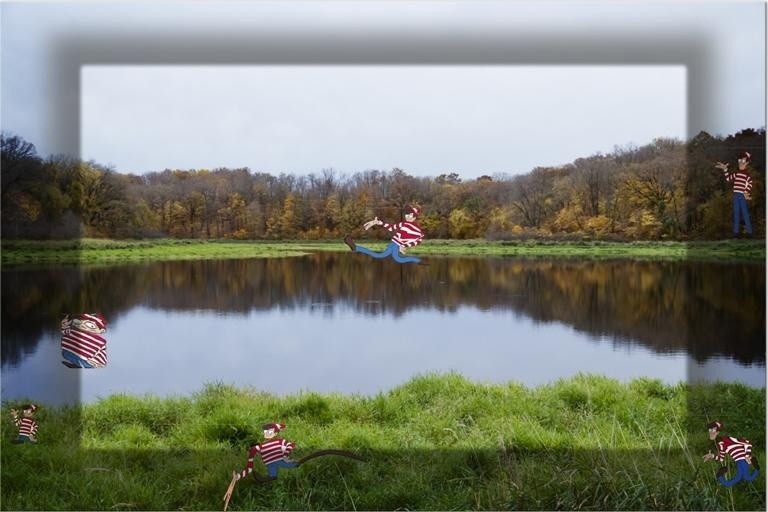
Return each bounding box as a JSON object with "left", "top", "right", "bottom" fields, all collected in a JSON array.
[
  {"left": 716, "top": 467, "right": 728, "bottom": 478},
  {"left": 750, "top": 457, "right": 761, "bottom": 470},
  {"left": 345, "top": 235, "right": 357, "bottom": 253}
]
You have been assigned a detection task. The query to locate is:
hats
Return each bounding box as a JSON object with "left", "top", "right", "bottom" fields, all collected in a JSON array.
[
  {"left": 271, "top": 422, "right": 287, "bottom": 432},
  {"left": 80, "top": 311, "right": 107, "bottom": 329},
  {"left": 405, "top": 204, "right": 423, "bottom": 217},
  {"left": 744, "top": 150, "right": 754, "bottom": 162},
  {"left": 30, "top": 403, "right": 38, "bottom": 411}
]
